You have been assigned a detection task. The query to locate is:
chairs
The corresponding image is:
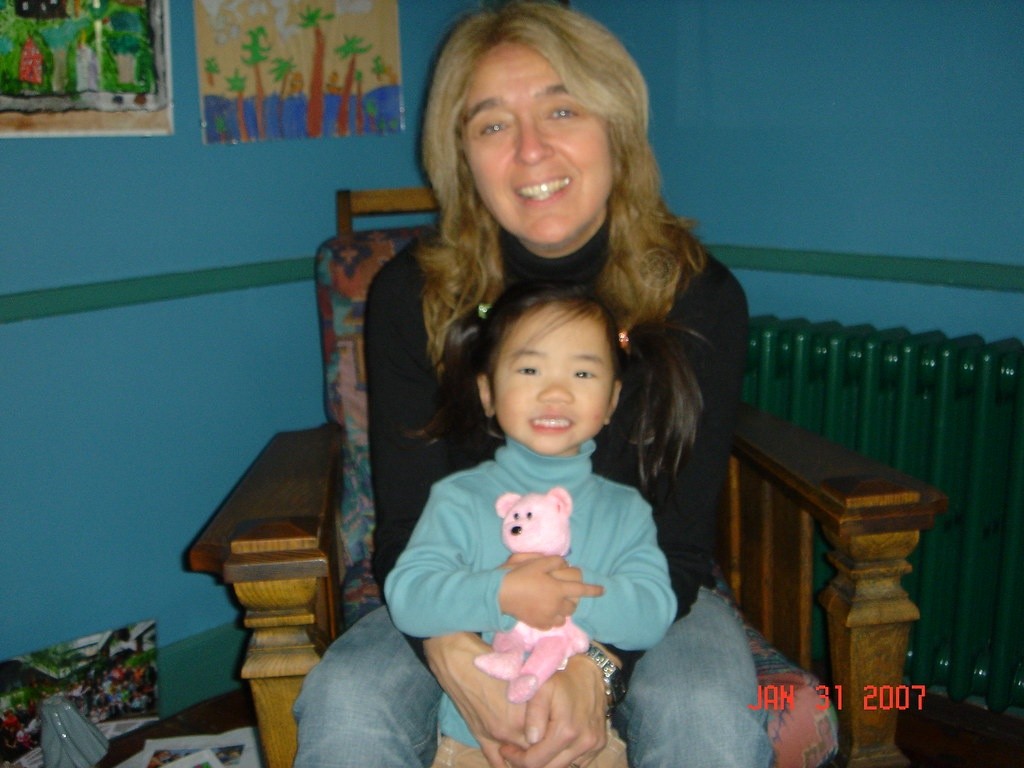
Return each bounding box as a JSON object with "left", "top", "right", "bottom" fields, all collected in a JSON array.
[{"left": 188, "top": 189, "right": 951, "bottom": 768}]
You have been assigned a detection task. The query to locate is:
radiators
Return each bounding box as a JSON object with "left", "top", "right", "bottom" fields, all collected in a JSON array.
[{"left": 739, "top": 315, "right": 1023, "bottom": 716}]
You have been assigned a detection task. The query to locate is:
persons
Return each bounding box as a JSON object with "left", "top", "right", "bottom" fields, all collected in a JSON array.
[
  {"left": 66, "top": 662, "right": 156, "bottom": 722},
  {"left": 291, "top": 0, "right": 773, "bottom": 768},
  {"left": 0, "top": 691, "right": 50, "bottom": 761},
  {"left": 384, "top": 283, "right": 678, "bottom": 768}
]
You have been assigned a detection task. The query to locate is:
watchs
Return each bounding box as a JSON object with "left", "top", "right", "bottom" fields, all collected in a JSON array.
[{"left": 584, "top": 643, "right": 627, "bottom": 719}]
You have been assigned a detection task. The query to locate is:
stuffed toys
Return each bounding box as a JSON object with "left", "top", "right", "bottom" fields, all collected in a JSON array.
[{"left": 476, "top": 487, "right": 590, "bottom": 706}]
